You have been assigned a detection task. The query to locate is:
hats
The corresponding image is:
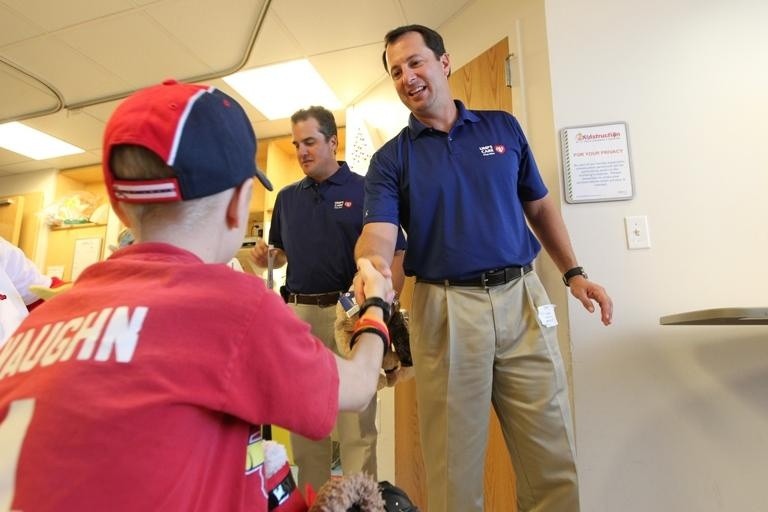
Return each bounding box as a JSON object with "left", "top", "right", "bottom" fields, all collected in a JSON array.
[{"left": 102, "top": 80, "right": 273, "bottom": 203}]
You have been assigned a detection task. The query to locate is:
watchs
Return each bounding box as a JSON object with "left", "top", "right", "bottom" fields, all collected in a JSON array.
[
  {"left": 358, "top": 296, "right": 394, "bottom": 325},
  {"left": 563, "top": 266, "right": 589, "bottom": 287}
]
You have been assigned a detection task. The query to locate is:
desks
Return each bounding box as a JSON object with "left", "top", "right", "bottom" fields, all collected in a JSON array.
[{"left": 659, "top": 307, "right": 768, "bottom": 325}]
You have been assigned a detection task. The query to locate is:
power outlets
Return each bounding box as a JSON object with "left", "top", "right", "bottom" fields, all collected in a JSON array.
[{"left": 625, "top": 215, "right": 651, "bottom": 249}]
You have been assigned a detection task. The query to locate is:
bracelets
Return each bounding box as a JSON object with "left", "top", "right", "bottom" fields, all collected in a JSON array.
[{"left": 349, "top": 316, "right": 390, "bottom": 359}]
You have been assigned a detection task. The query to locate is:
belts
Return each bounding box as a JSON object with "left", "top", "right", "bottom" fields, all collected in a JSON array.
[
  {"left": 289, "top": 290, "right": 349, "bottom": 307},
  {"left": 416, "top": 264, "right": 532, "bottom": 289}
]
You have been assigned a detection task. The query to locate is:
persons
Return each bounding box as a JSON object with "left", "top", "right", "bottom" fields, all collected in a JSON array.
[
  {"left": 250, "top": 105, "right": 408, "bottom": 508},
  {"left": 0, "top": 78, "right": 398, "bottom": 511},
  {"left": 351, "top": 24, "right": 614, "bottom": 512},
  {"left": 1, "top": 237, "right": 73, "bottom": 312}
]
